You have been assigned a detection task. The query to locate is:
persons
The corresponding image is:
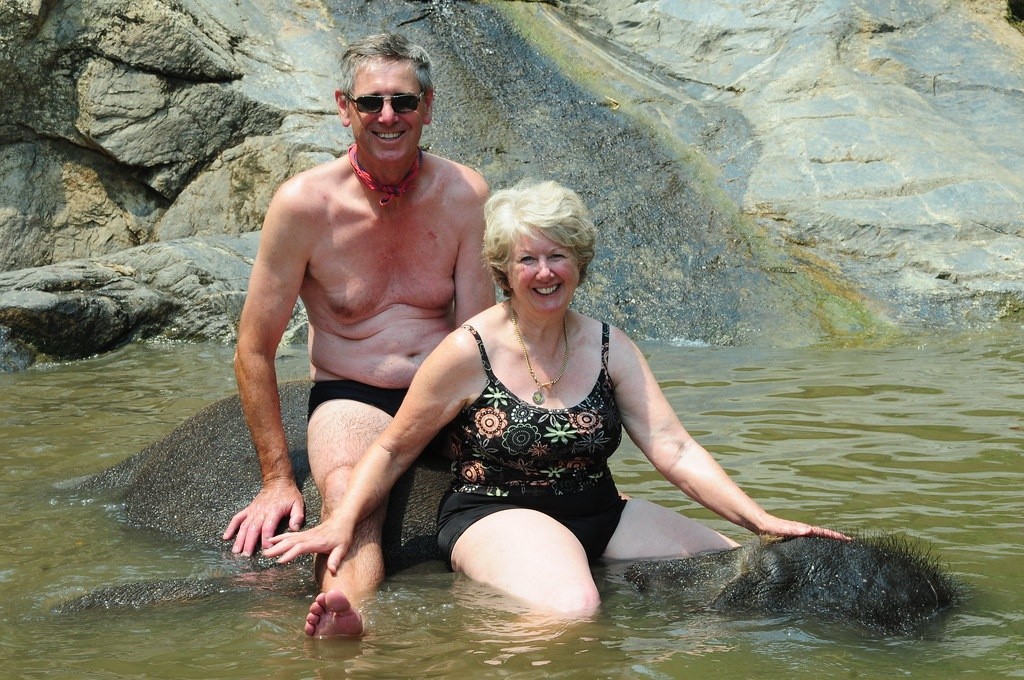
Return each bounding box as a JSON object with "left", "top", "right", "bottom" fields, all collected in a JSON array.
[
  {"left": 232, "top": 34, "right": 496, "bottom": 640},
  {"left": 259, "top": 180, "right": 855, "bottom": 635}
]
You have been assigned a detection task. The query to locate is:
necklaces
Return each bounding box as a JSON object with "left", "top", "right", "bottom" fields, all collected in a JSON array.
[{"left": 508, "top": 299, "right": 570, "bottom": 403}]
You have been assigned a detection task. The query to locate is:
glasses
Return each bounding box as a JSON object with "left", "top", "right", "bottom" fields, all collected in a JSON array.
[{"left": 345, "top": 89, "right": 423, "bottom": 114}]
